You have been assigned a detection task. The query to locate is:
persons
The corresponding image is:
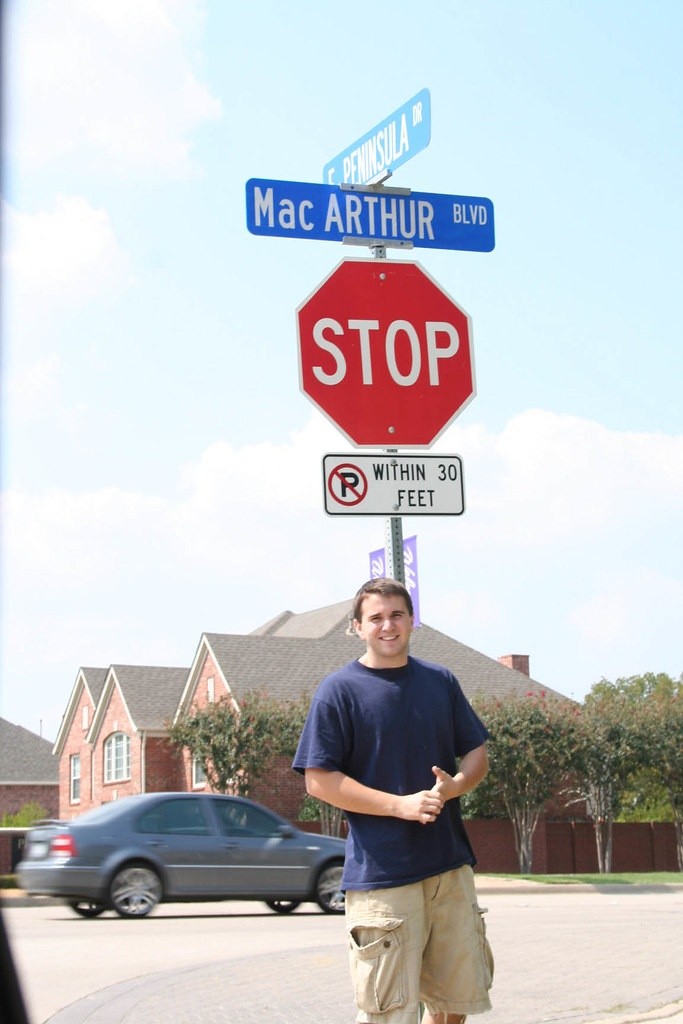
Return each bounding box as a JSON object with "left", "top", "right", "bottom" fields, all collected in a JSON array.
[{"left": 291, "top": 578, "right": 495, "bottom": 1024}]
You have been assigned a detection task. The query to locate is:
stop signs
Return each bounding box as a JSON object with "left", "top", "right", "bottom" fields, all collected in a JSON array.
[{"left": 293, "top": 256, "right": 477, "bottom": 451}]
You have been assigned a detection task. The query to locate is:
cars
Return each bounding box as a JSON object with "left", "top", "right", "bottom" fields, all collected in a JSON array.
[{"left": 13, "top": 791, "right": 348, "bottom": 920}]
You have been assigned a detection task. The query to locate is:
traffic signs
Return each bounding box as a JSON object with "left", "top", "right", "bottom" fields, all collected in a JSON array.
[
  {"left": 244, "top": 177, "right": 496, "bottom": 254},
  {"left": 317, "top": 87, "right": 434, "bottom": 184}
]
